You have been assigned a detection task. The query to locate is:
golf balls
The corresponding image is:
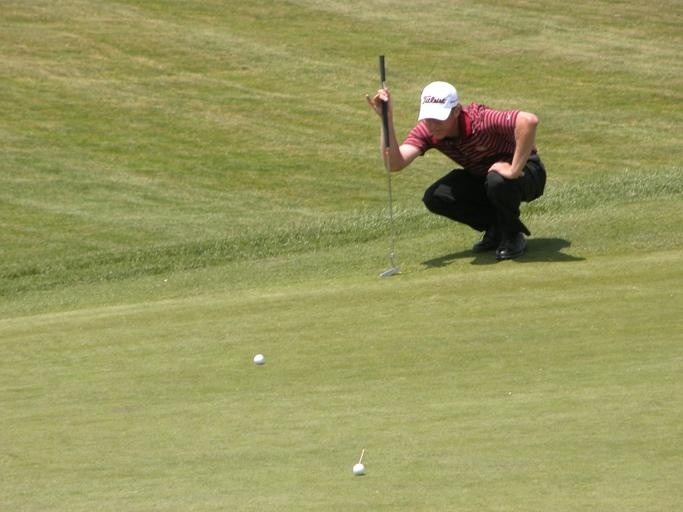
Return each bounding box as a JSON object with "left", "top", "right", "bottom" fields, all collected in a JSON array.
[
  {"left": 352, "top": 464, "right": 365, "bottom": 476},
  {"left": 254, "top": 354, "right": 265, "bottom": 366}
]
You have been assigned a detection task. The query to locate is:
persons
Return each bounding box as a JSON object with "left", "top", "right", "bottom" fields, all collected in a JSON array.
[{"left": 365, "top": 80, "right": 547, "bottom": 261}]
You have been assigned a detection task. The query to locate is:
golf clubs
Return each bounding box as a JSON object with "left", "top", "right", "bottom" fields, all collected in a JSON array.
[{"left": 378, "top": 55, "right": 402, "bottom": 277}]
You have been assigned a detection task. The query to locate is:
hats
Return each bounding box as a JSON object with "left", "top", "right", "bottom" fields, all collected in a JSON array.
[{"left": 417, "top": 81, "right": 459, "bottom": 121}]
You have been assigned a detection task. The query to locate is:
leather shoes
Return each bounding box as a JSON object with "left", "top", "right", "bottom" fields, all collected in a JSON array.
[
  {"left": 496, "top": 232, "right": 527, "bottom": 259},
  {"left": 473, "top": 232, "right": 499, "bottom": 251}
]
